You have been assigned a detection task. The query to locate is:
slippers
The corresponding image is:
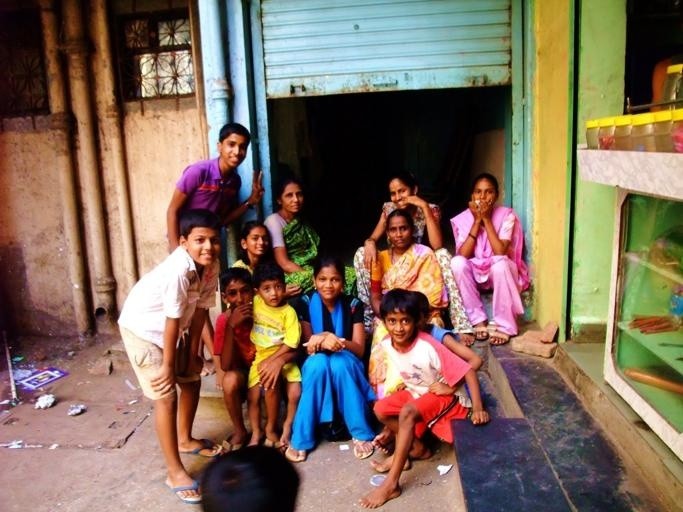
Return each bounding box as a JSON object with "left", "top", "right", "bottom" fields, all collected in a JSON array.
[
  {"left": 164, "top": 479, "right": 203, "bottom": 504},
  {"left": 352, "top": 438, "right": 374, "bottom": 459},
  {"left": 219, "top": 434, "right": 247, "bottom": 457},
  {"left": 264, "top": 438, "right": 284, "bottom": 455},
  {"left": 475, "top": 327, "right": 489, "bottom": 341},
  {"left": 285, "top": 444, "right": 306, "bottom": 463},
  {"left": 489, "top": 329, "right": 510, "bottom": 346},
  {"left": 181, "top": 439, "right": 223, "bottom": 457}
]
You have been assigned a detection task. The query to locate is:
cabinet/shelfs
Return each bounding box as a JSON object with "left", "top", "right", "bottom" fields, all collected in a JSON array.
[{"left": 601, "top": 185, "right": 683, "bottom": 463}]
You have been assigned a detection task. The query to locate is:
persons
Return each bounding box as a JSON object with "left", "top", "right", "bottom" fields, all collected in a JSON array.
[{"left": 116, "top": 122, "right": 531, "bottom": 512}]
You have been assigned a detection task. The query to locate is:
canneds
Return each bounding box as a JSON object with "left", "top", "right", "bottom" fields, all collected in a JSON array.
[{"left": 586, "top": 63, "right": 683, "bottom": 153}]
